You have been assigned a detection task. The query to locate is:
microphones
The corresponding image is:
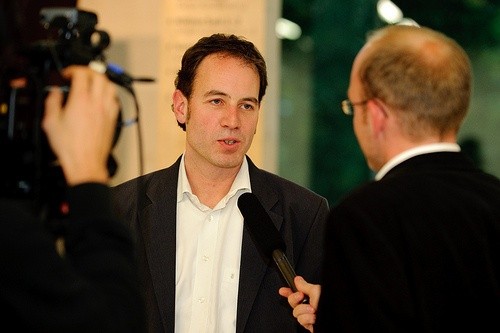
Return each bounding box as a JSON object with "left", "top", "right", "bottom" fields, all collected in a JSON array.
[{"left": 238, "top": 192, "right": 309, "bottom": 305}]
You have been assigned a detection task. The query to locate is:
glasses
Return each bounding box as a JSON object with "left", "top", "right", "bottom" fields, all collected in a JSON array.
[{"left": 342, "top": 98, "right": 388, "bottom": 117}]
